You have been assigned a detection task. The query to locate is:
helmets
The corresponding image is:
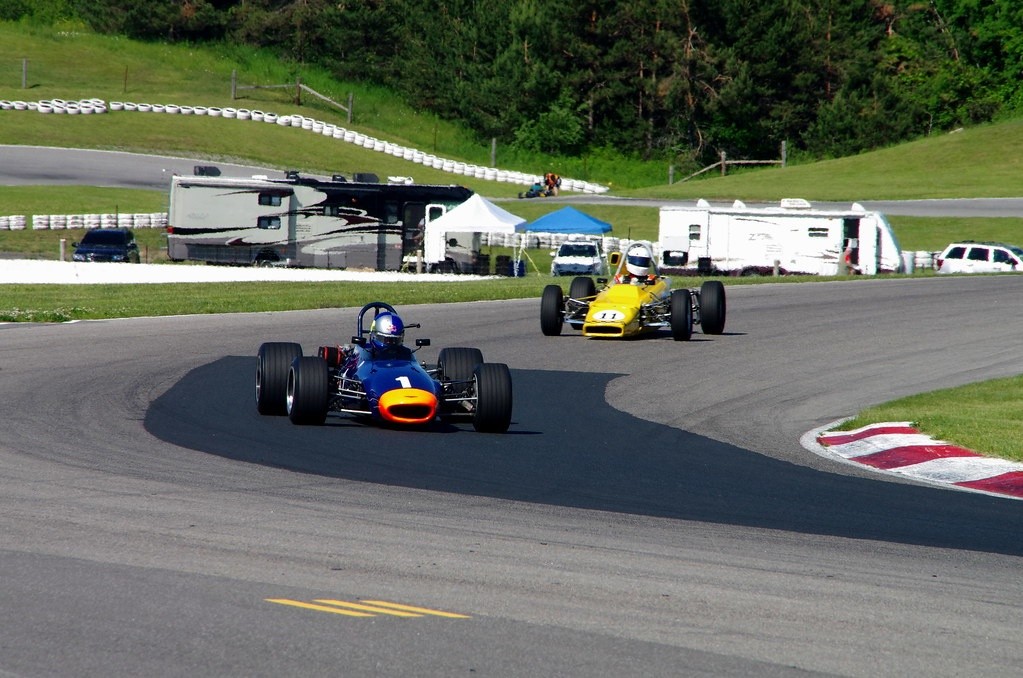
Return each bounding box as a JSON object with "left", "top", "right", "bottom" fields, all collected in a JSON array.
[
  {"left": 368, "top": 312, "right": 404, "bottom": 353},
  {"left": 625, "top": 244, "right": 651, "bottom": 276}
]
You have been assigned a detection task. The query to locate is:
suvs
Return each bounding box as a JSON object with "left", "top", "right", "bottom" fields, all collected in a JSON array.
[
  {"left": 71, "top": 227, "right": 141, "bottom": 263},
  {"left": 935, "top": 238, "right": 1022, "bottom": 274}
]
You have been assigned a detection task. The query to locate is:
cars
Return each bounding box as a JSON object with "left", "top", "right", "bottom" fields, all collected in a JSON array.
[{"left": 549, "top": 240, "right": 608, "bottom": 276}]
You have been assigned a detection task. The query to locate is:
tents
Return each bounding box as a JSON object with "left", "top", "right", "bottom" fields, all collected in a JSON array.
[
  {"left": 513, "top": 206, "right": 620, "bottom": 277},
  {"left": 424, "top": 193, "right": 528, "bottom": 273}
]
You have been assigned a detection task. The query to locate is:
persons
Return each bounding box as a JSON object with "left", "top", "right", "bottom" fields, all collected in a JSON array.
[
  {"left": 531, "top": 179, "right": 547, "bottom": 197},
  {"left": 544, "top": 172, "right": 561, "bottom": 198},
  {"left": 341, "top": 313, "right": 407, "bottom": 377},
  {"left": 614, "top": 246, "right": 656, "bottom": 286}
]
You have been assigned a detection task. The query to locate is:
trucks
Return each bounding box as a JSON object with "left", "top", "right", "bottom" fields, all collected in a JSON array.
[
  {"left": 164, "top": 164, "right": 483, "bottom": 275},
  {"left": 656, "top": 198, "right": 905, "bottom": 278}
]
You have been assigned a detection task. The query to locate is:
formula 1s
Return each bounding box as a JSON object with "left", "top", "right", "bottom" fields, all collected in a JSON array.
[
  {"left": 252, "top": 299, "right": 516, "bottom": 433},
  {"left": 538, "top": 241, "right": 728, "bottom": 343}
]
